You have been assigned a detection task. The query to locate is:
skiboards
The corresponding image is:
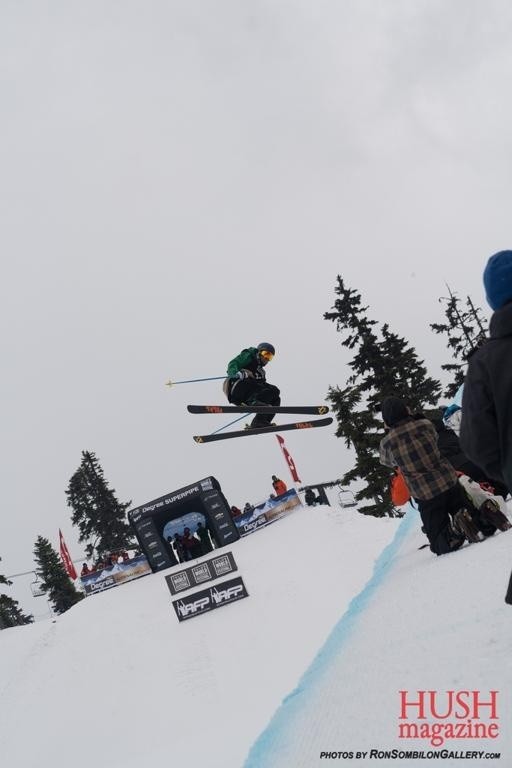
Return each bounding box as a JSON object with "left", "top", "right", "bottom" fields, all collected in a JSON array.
[{"left": 188, "top": 405, "right": 333, "bottom": 443}]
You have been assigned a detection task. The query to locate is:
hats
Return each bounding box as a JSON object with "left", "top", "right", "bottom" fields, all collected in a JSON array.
[
  {"left": 483, "top": 250, "right": 512, "bottom": 311},
  {"left": 257, "top": 342, "right": 275, "bottom": 355},
  {"left": 382, "top": 398, "right": 409, "bottom": 428}
]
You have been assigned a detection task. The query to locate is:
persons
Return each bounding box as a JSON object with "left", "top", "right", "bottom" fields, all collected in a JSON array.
[
  {"left": 457, "top": 249, "right": 511, "bottom": 494},
  {"left": 377, "top": 396, "right": 512, "bottom": 558},
  {"left": 223, "top": 342, "right": 281, "bottom": 429},
  {"left": 80, "top": 472, "right": 287, "bottom": 578},
  {"left": 303, "top": 485, "right": 324, "bottom": 508}
]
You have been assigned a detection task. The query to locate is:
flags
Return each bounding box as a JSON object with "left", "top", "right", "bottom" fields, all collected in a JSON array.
[
  {"left": 276, "top": 433, "right": 301, "bottom": 483},
  {"left": 58, "top": 529, "right": 78, "bottom": 580}
]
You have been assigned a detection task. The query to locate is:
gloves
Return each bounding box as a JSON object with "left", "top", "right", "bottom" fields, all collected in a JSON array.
[{"left": 237, "top": 368, "right": 255, "bottom": 383}]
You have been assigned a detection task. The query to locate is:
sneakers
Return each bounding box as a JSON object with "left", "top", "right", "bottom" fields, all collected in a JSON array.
[
  {"left": 453, "top": 508, "right": 485, "bottom": 543},
  {"left": 251, "top": 419, "right": 276, "bottom": 428},
  {"left": 479, "top": 497, "right": 512, "bottom": 532},
  {"left": 241, "top": 398, "right": 272, "bottom": 407}
]
